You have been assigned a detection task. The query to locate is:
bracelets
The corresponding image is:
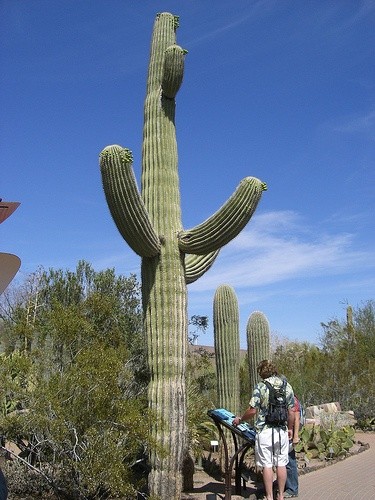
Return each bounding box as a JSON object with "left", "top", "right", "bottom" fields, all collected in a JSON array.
[{"left": 238, "top": 418, "right": 243, "bottom": 424}]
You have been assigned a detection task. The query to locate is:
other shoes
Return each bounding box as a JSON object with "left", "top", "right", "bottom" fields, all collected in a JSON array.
[{"left": 282, "top": 490, "right": 298, "bottom": 498}]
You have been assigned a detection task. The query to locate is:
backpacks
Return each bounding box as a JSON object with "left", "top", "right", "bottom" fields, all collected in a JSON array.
[{"left": 260, "top": 380, "right": 287, "bottom": 424}]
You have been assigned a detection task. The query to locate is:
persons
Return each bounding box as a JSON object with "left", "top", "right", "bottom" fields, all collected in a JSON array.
[
  {"left": 234, "top": 359, "right": 296, "bottom": 500},
  {"left": 278, "top": 373, "right": 302, "bottom": 499}
]
